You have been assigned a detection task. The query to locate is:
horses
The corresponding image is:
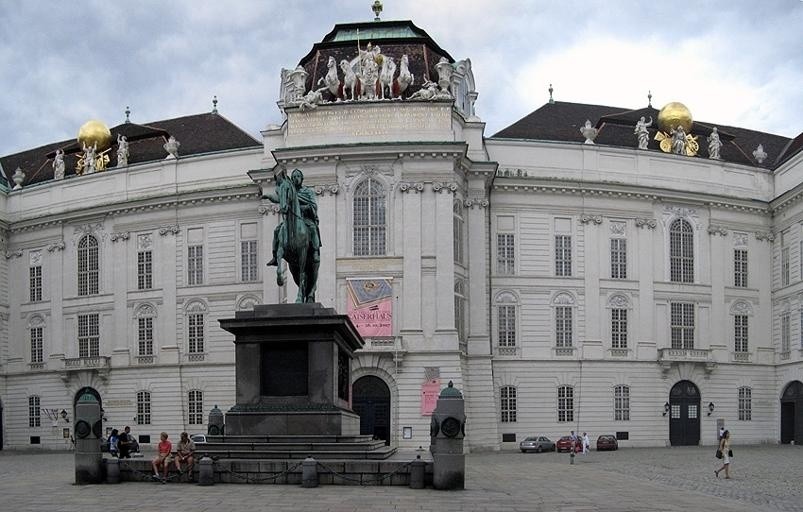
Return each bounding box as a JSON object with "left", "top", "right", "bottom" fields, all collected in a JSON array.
[
  {"left": 274, "top": 177, "right": 320, "bottom": 304},
  {"left": 314, "top": 54, "right": 340, "bottom": 100},
  {"left": 339, "top": 59, "right": 358, "bottom": 102},
  {"left": 395, "top": 54, "right": 415, "bottom": 99},
  {"left": 378, "top": 56, "right": 397, "bottom": 101}
]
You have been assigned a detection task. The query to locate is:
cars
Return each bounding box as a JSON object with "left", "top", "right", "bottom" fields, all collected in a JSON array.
[
  {"left": 519, "top": 433, "right": 622, "bottom": 453},
  {"left": 100, "top": 435, "right": 142, "bottom": 455}
]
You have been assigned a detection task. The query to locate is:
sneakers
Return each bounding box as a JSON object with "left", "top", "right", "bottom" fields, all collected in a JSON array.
[
  {"left": 152, "top": 468, "right": 193, "bottom": 484},
  {"left": 714, "top": 470, "right": 733, "bottom": 480}
]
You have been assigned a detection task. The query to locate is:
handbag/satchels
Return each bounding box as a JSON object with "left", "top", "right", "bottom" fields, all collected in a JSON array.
[
  {"left": 715, "top": 449, "right": 723, "bottom": 459},
  {"left": 728, "top": 449, "right": 734, "bottom": 458}
]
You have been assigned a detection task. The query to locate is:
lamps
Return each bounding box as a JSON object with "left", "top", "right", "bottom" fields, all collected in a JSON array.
[
  {"left": 99, "top": 407, "right": 107, "bottom": 422},
  {"left": 706, "top": 401, "right": 716, "bottom": 417},
  {"left": 662, "top": 400, "right": 671, "bottom": 416},
  {"left": 59, "top": 409, "right": 71, "bottom": 422}
]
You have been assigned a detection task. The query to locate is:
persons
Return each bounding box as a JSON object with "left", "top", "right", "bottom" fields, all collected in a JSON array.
[
  {"left": 633, "top": 114, "right": 653, "bottom": 150},
  {"left": 149, "top": 431, "right": 173, "bottom": 484},
  {"left": 714, "top": 430, "right": 731, "bottom": 479},
  {"left": 669, "top": 125, "right": 689, "bottom": 156},
  {"left": 568, "top": 431, "right": 578, "bottom": 455},
  {"left": 580, "top": 431, "right": 591, "bottom": 453},
  {"left": 51, "top": 149, "right": 66, "bottom": 180},
  {"left": 718, "top": 427, "right": 724, "bottom": 446},
  {"left": 115, "top": 133, "right": 128, "bottom": 168},
  {"left": 81, "top": 139, "right": 97, "bottom": 175},
  {"left": 704, "top": 125, "right": 724, "bottom": 161},
  {"left": 172, "top": 432, "right": 195, "bottom": 478},
  {"left": 118, "top": 425, "right": 136, "bottom": 458},
  {"left": 107, "top": 427, "right": 118, "bottom": 456},
  {"left": 355, "top": 41, "right": 381, "bottom": 74},
  {"left": 266, "top": 167, "right": 323, "bottom": 304}
]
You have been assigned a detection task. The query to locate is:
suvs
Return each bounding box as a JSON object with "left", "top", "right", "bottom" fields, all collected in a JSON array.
[{"left": 189, "top": 432, "right": 208, "bottom": 444}]
